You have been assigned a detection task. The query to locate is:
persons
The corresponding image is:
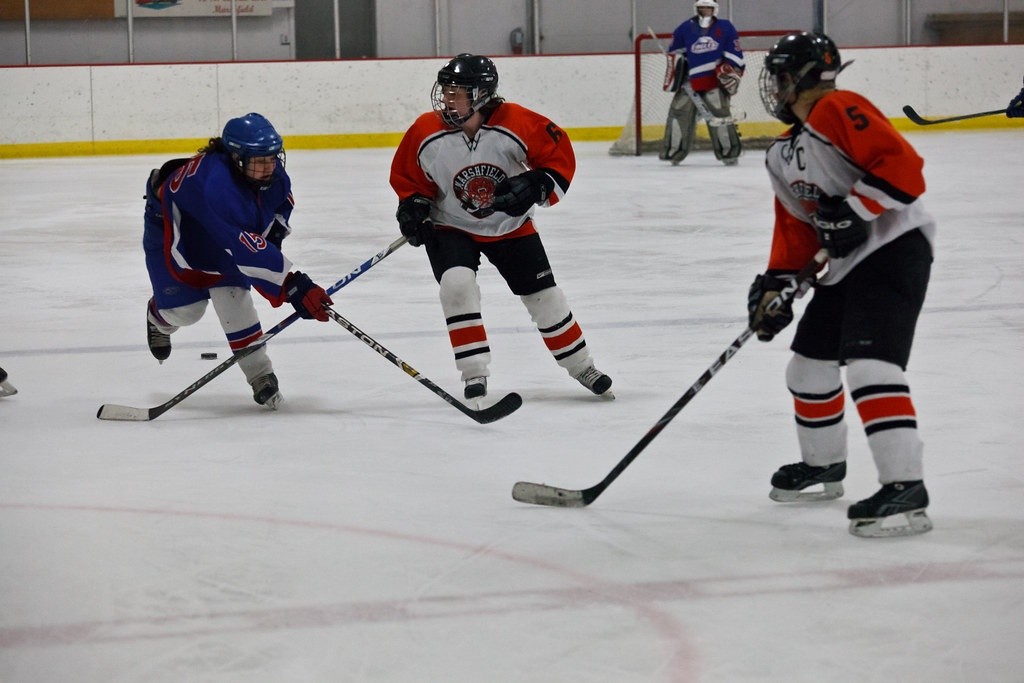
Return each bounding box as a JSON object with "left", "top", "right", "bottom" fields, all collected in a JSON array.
[
  {"left": 660, "top": 0, "right": 745, "bottom": 166},
  {"left": 390, "top": 53, "right": 614, "bottom": 407},
  {"left": 141, "top": 112, "right": 333, "bottom": 410},
  {"left": 748, "top": 32, "right": 935, "bottom": 537}
]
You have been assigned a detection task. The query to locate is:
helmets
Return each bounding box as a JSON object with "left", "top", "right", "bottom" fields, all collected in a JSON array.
[
  {"left": 430, "top": 52, "right": 499, "bottom": 128},
  {"left": 222, "top": 112, "right": 286, "bottom": 192},
  {"left": 693, "top": 0, "right": 719, "bottom": 28},
  {"left": 758, "top": 31, "right": 841, "bottom": 125}
]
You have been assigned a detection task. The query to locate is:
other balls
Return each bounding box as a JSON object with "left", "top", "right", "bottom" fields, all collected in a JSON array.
[{"left": 200, "top": 352, "right": 217, "bottom": 360}]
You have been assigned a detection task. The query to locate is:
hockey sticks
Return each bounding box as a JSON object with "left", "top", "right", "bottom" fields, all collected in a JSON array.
[
  {"left": 326, "top": 305, "right": 523, "bottom": 425},
  {"left": 646, "top": 25, "right": 748, "bottom": 128},
  {"left": 95, "top": 236, "right": 410, "bottom": 423},
  {"left": 510, "top": 249, "right": 829, "bottom": 508},
  {"left": 902, "top": 103, "right": 1008, "bottom": 126}
]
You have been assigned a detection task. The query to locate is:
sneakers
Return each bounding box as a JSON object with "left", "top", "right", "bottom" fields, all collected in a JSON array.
[
  {"left": 250, "top": 371, "right": 284, "bottom": 410},
  {"left": 769, "top": 460, "right": 846, "bottom": 503},
  {"left": 847, "top": 480, "right": 934, "bottom": 539},
  {"left": 575, "top": 364, "right": 617, "bottom": 400},
  {"left": 146, "top": 299, "right": 171, "bottom": 365},
  {"left": 464, "top": 377, "right": 488, "bottom": 412}
]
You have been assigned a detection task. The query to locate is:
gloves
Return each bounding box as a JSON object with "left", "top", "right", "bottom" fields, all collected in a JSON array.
[
  {"left": 814, "top": 191, "right": 868, "bottom": 259},
  {"left": 1006, "top": 87, "right": 1024, "bottom": 118},
  {"left": 396, "top": 192, "right": 435, "bottom": 247},
  {"left": 284, "top": 269, "right": 333, "bottom": 322},
  {"left": 747, "top": 267, "right": 802, "bottom": 342},
  {"left": 493, "top": 168, "right": 555, "bottom": 217}
]
